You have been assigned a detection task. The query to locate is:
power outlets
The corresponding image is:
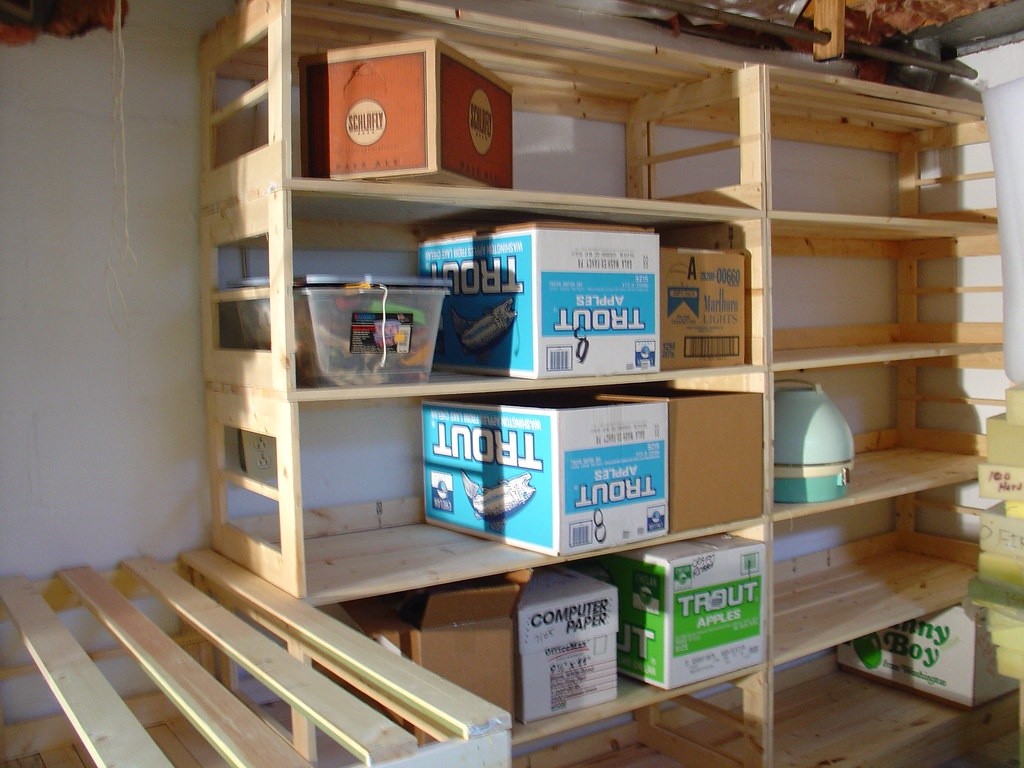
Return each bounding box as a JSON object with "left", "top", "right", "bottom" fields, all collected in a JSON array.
[{"left": 239, "top": 429, "right": 278, "bottom": 480}]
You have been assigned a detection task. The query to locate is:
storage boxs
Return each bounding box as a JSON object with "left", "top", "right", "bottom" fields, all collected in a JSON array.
[
  {"left": 424, "top": 396, "right": 670, "bottom": 562},
  {"left": 835, "top": 382, "right": 1024, "bottom": 709},
  {"left": 551, "top": 531, "right": 767, "bottom": 691},
  {"left": 590, "top": 391, "right": 764, "bottom": 534},
  {"left": 408, "top": 216, "right": 662, "bottom": 380},
  {"left": 298, "top": 38, "right": 515, "bottom": 191},
  {"left": 661, "top": 246, "right": 750, "bottom": 371},
  {"left": 221, "top": 274, "right": 454, "bottom": 388},
  {"left": 513, "top": 563, "right": 620, "bottom": 726},
  {"left": 345, "top": 567, "right": 534, "bottom": 743}
]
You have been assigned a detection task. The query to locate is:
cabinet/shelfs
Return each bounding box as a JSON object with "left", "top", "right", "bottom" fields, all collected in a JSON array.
[{"left": 192, "top": 1, "right": 1024, "bottom": 768}]
